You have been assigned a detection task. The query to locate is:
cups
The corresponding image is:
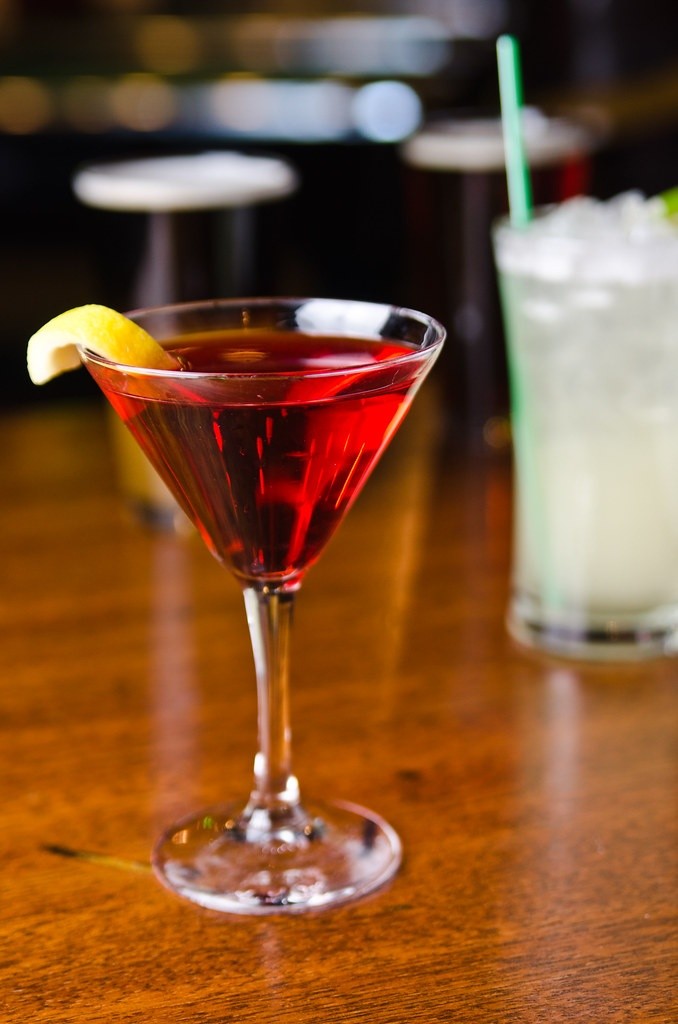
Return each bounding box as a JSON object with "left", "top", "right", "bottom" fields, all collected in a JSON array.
[
  {"left": 70, "top": 156, "right": 300, "bottom": 540},
  {"left": 398, "top": 113, "right": 590, "bottom": 478},
  {"left": 484, "top": 199, "right": 678, "bottom": 664}
]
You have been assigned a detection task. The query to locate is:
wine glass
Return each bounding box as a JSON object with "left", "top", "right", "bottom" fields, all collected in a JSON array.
[{"left": 74, "top": 295, "right": 448, "bottom": 916}]
